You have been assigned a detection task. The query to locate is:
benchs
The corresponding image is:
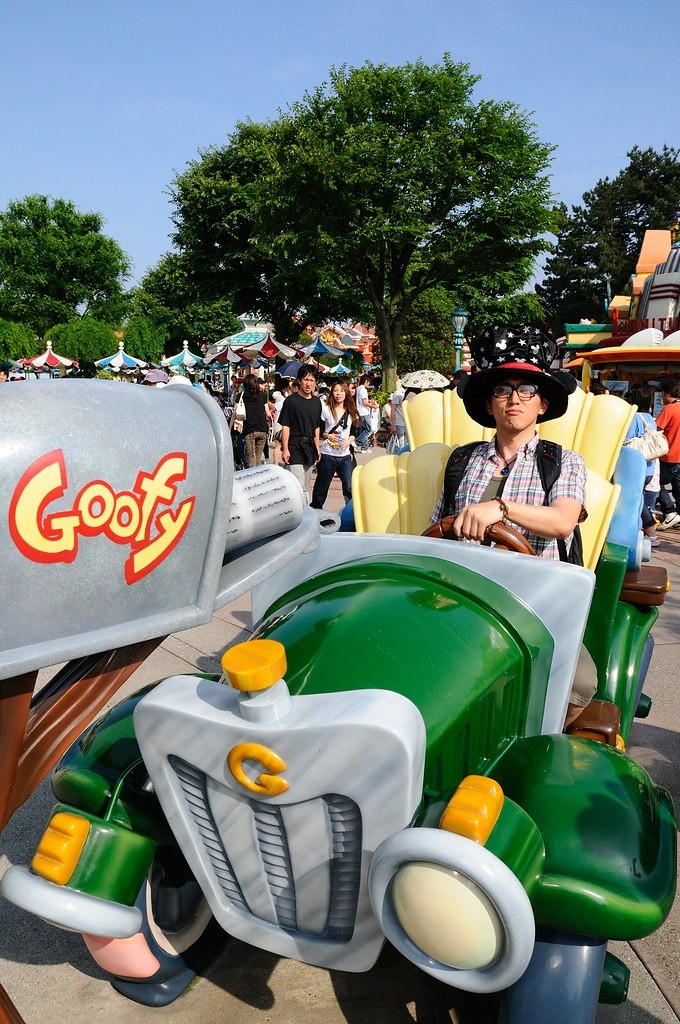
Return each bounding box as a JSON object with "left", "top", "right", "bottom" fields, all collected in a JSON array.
[
  {"left": 351, "top": 444, "right": 622, "bottom": 571},
  {"left": 402, "top": 385, "right": 638, "bottom": 480}
]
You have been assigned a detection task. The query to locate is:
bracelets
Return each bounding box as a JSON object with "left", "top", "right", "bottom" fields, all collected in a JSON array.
[
  {"left": 492, "top": 496, "right": 510, "bottom": 525},
  {"left": 326, "top": 433, "right": 329, "bottom": 438}
]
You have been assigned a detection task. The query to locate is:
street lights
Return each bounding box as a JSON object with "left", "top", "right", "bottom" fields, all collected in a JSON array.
[{"left": 451, "top": 305, "right": 472, "bottom": 372}]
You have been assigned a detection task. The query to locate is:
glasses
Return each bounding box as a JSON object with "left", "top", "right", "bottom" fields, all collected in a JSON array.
[{"left": 488, "top": 383, "right": 539, "bottom": 398}]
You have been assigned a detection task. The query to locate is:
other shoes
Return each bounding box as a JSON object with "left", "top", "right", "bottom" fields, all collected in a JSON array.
[
  {"left": 361, "top": 449, "right": 372, "bottom": 454},
  {"left": 353, "top": 447, "right": 358, "bottom": 452}
]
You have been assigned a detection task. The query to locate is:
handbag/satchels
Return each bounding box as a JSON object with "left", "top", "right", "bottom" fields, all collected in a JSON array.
[
  {"left": 624, "top": 413, "right": 669, "bottom": 463},
  {"left": 235, "top": 391, "right": 247, "bottom": 421},
  {"left": 386, "top": 434, "right": 404, "bottom": 455}
]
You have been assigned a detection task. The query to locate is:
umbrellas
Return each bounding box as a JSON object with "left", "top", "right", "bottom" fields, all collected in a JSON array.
[
  {"left": 23, "top": 333, "right": 359, "bottom": 371},
  {"left": 401, "top": 370, "right": 450, "bottom": 389}
]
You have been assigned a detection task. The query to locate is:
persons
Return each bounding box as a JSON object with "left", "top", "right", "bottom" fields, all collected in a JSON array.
[
  {"left": 429, "top": 325, "right": 597, "bottom": 734},
  {"left": 0, "top": 367, "right": 9, "bottom": 383},
  {"left": 622, "top": 383, "right": 661, "bottom": 548},
  {"left": 104, "top": 369, "right": 680, "bottom": 472},
  {"left": 309, "top": 382, "right": 357, "bottom": 510},
  {"left": 278, "top": 365, "right": 322, "bottom": 507},
  {"left": 654, "top": 377, "right": 680, "bottom": 529}
]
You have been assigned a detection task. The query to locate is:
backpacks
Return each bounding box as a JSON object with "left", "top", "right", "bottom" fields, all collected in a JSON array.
[{"left": 443, "top": 439, "right": 584, "bottom": 567}]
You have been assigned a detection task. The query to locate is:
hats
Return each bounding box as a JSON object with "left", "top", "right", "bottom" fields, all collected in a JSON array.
[
  {"left": 387, "top": 394, "right": 394, "bottom": 401},
  {"left": 140, "top": 370, "right": 169, "bottom": 384},
  {"left": 462, "top": 323, "right": 569, "bottom": 428}
]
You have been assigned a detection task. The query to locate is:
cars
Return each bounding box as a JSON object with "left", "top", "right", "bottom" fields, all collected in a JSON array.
[{"left": 0, "top": 377, "right": 680, "bottom": 1023}]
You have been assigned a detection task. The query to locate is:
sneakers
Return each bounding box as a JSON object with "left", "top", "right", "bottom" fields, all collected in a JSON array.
[
  {"left": 644, "top": 535, "right": 661, "bottom": 547},
  {"left": 657, "top": 515, "right": 680, "bottom": 531}
]
